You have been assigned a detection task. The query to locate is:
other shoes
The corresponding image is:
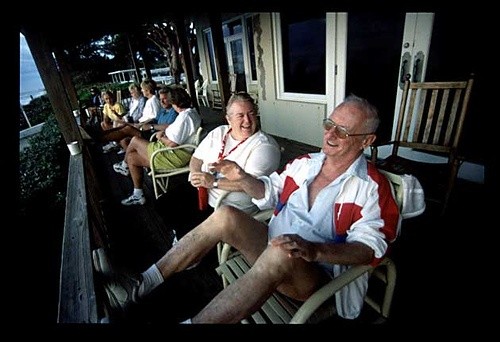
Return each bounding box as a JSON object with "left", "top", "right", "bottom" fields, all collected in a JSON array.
[{"left": 117, "top": 148, "right": 126, "bottom": 154}]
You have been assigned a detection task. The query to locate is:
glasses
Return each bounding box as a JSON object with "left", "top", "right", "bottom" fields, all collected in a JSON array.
[{"left": 324, "top": 118, "right": 374, "bottom": 140}]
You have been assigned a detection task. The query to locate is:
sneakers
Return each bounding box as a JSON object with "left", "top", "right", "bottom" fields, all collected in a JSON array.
[
  {"left": 92, "top": 247, "right": 141, "bottom": 309},
  {"left": 121, "top": 194, "right": 146, "bottom": 206},
  {"left": 113, "top": 162, "right": 130, "bottom": 176}
]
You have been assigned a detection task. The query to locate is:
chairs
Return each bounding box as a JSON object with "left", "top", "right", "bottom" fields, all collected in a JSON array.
[
  {"left": 216, "top": 167, "right": 403, "bottom": 324},
  {"left": 147, "top": 123, "right": 204, "bottom": 199},
  {"left": 366, "top": 72, "right": 476, "bottom": 208},
  {"left": 185, "top": 79, "right": 210, "bottom": 107}
]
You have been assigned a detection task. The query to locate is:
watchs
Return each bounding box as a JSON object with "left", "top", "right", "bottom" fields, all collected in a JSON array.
[
  {"left": 139, "top": 125, "right": 143, "bottom": 132},
  {"left": 213, "top": 178, "right": 218, "bottom": 188},
  {"left": 150, "top": 124, "right": 154, "bottom": 130}
]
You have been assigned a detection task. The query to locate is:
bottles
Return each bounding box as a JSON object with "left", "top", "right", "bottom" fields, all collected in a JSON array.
[{"left": 199, "top": 186, "right": 208, "bottom": 210}]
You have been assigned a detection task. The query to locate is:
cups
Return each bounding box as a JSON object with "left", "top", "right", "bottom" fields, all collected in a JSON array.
[
  {"left": 73, "top": 110, "right": 78, "bottom": 118},
  {"left": 67, "top": 141, "right": 81, "bottom": 156}
]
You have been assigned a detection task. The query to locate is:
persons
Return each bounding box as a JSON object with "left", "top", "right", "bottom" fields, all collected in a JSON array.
[
  {"left": 92, "top": 94, "right": 402, "bottom": 324},
  {"left": 98, "top": 90, "right": 106, "bottom": 108},
  {"left": 91, "top": 86, "right": 178, "bottom": 144},
  {"left": 100, "top": 91, "right": 124, "bottom": 152},
  {"left": 120, "top": 78, "right": 160, "bottom": 151},
  {"left": 108, "top": 87, "right": 115, "bottom": 95},
  {"left": 86, "top": 87, "right": 100, "bottom": 126},
  {"left": 118, "top": 82, "right": 144, "bottom": 154},
  {"left": 112, "top": 87, "right": 202, "bottom": 206},
  {"left": 168, "top": 91, "right": 282, "bottom": 270}
]
